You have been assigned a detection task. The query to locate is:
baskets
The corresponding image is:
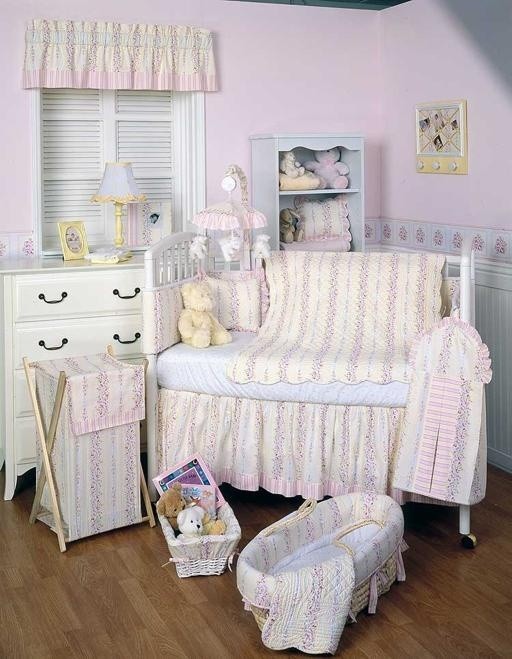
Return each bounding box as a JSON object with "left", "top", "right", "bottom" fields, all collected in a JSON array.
[
  {"left": 156, "top": 484, "right": 241, "bottom": 580},
  {"left": 236, "top": 489, "right": 404, "bottom": 633}
]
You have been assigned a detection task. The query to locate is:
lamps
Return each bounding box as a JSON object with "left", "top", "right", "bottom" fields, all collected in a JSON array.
[{"left": 90, "top": 160, "right": 146, "bottom": 262}]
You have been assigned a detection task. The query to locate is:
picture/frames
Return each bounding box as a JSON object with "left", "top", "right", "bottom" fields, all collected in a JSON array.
[
  {"left": 414, "top": 100, "right": 468, "bottom": 176},
  {"left": 58, "top": 220, "right": 89, "bottom": 261}
]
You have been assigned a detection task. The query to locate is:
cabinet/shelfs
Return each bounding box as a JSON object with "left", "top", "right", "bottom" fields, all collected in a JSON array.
[
  {"left": 248, "top": 132, "right": 365, "bottom": 253},
  {"left": 0, "top": 260, "right": 146, "bottom": 502}
]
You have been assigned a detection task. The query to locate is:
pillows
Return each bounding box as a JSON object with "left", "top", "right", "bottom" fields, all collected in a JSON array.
[
  {"left": 198, "top": 269, "right": 271, "bottom": 334},
  {"left": 294, "top": 197, "right": 352, "bottom": 242}
]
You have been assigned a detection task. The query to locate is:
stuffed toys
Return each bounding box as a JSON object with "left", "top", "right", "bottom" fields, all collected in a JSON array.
[
  {"left": 157, "top": 482, "right": 226, "bottom": 535},
  {"left": 279, "top": 151, "right": 306, "bottom": 179},
  {"left": 189, "top": 231, "right": 271, "bottom": 262},
  {"left": 280, "top": 206, "right": 304, "bottom": 243},
  {"left": 303, "top": 147, "right": 349, "bottom": 189},
  {"left": 176, "top": 506, "right": 205, "bottom": 538}
]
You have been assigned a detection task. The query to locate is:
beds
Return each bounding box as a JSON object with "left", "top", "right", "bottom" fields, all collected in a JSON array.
[{"left": 144, "top": 231, "right": 479, "bottom": 549}]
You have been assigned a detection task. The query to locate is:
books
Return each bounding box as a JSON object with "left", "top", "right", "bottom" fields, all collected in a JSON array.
[
  {"left": 90, "top": 251, "right": 132, "bottom": 264},
  {"left": 152, "top": 451, "right": 226, "bottom": 510},
  {"left": 179, "top": 483, "right": 217, "bottom": 515},
  {"left": 178, "top": 283, "right": 233, "bottom": 348},
  {"left": 84, "top": 247, "right": 126, "bottom": 260}
]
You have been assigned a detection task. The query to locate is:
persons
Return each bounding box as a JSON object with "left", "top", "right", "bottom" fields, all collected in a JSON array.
[
  {"left": 150, "top": 214, "right": 159, "bottom": 223},
  {"left": 68, "top": 233, "right": 80, "bottom": 250},
  {"left": 421, "top": 113, "right": 458, "bottom": 149}
]
